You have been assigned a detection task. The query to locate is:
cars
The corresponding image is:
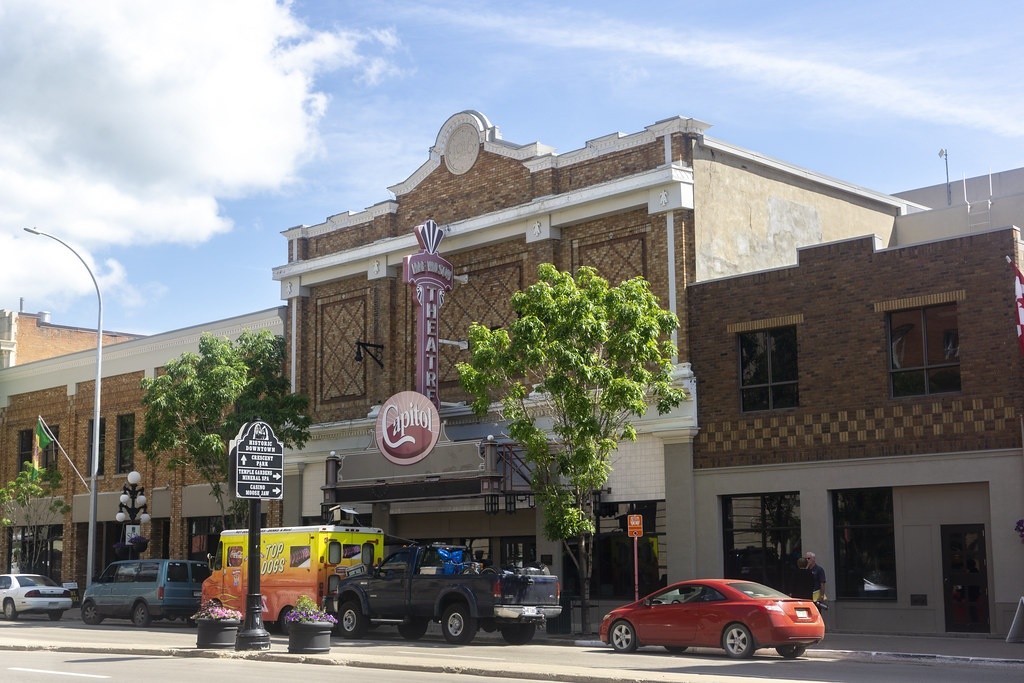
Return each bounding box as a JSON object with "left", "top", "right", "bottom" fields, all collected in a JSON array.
[
  {"left": 598, "top": 578, "right": 825, "bottom": 659},
  {"left": 0, "top": 573, "right": 73, "bottom": 621}
]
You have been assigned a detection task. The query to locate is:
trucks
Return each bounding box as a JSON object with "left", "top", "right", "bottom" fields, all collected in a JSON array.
[{"left": 200, "top": 525, "right": 384, "bottom": 635}]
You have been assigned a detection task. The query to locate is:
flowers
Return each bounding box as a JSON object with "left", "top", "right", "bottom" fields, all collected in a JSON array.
[
  {"left": 283, "top": 595, "right": 338, "bottom": 627},
  {"left": 127, "top": 532, "right": 149, "bottom": 543},
  {"left": 113, "top": 542, "right": 124, "bottom": 548},
  {"left": 190, "top": 594, "right": 243, "bottom": 625}
]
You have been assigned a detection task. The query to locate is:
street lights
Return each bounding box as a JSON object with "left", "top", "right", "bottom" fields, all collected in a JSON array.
[
  {"left": 115, "top": 470, "right": 151, "bottom": 560},
  {"left": 24, "top": 227, "right": 104, "bottom": 587}
]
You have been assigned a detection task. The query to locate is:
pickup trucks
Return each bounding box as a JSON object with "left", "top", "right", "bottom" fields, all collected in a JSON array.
[{"left": 327, "top": 544, "right": 563, "bottom": 644}]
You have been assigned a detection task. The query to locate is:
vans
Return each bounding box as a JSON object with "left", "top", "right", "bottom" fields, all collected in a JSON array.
[{"left": 79, "top": 559, "right": 211, "bottom": 627}]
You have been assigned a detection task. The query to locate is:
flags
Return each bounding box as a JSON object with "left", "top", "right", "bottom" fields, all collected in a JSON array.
[{"left": 35, "top": 415, "right": 53, "bottom": 455}]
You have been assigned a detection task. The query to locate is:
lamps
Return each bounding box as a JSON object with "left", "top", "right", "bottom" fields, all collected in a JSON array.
[{"left": 354, "top": 340, "right": 384, "bottom": 369}]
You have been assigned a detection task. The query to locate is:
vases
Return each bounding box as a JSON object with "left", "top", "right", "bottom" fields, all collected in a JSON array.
[
  {"left": 196, "top": 618, "right": 241, "bottom": 649},
  {"left": 286, "top": 621, "right": 334, "bottom": 654},
  {"left": 132, "top": 542, "right": 147, "bottom": 553},
  {"left": 114, "top": 548, "right": 125, "bottom": 554}
]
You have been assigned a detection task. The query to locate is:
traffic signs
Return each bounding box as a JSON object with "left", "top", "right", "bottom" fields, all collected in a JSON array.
[{"left": 234, "top": 422, "right": 285, "bottom": 500}]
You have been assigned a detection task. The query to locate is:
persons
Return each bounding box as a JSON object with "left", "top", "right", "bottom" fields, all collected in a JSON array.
[
  {"left": 473, "top": 550, "right": 489, "bottom": 568},
  {"left": 788, "top": 552, "right": 826, "bottom": 643}
]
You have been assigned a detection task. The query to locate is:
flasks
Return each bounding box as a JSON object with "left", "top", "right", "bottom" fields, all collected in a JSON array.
[{"left": 816, "top": 601, "right": 828, "bottom": 610}]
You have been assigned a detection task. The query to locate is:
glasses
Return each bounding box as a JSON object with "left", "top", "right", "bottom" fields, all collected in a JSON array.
[{"left": 806, "top": 557, "right": 810, "bottom": 560}]
